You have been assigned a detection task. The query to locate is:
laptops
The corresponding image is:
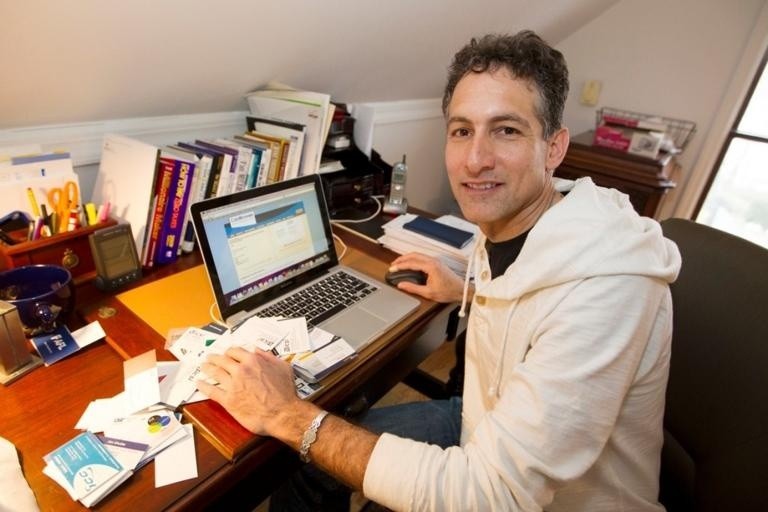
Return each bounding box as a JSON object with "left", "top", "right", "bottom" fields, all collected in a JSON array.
[{"left": 189, "top": 172, "right": 423, "bottom": 385}]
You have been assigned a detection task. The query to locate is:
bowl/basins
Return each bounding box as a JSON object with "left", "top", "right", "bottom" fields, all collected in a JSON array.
[{"left": 0, "top": 262, "right": 77, "bottom": 337}]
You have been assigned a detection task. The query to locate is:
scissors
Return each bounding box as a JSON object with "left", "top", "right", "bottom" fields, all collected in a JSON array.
[{"left": 48, "top": 181, "right": 79, "bottom": 230}]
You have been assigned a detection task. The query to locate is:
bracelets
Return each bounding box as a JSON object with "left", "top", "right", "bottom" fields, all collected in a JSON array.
[{"left": 298, "top": 409, "right": 331, "bottom": 465}]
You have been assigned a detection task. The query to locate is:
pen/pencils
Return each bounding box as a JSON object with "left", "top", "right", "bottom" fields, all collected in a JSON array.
[{"left": 26, "top": 187, "right": 111, "bottom": 241}]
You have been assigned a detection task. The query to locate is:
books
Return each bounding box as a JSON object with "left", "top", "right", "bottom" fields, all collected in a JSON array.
[
  {"left": 403, "top": 216, "right": 474, "bottom": 250},
  {"left": 88, "top": 81, "right": 335, "bottom": 270}
]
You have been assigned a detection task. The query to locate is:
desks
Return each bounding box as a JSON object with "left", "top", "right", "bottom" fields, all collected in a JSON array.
[{"left": 0, "top": 206, "right": 468, "bottom": 512}]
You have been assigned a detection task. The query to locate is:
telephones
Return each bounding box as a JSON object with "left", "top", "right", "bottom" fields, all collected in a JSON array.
[{"left": 383, "top": 154, "right": 408, "bottom": 214}]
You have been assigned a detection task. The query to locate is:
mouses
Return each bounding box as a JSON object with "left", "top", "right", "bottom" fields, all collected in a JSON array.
[{"left": 384, "top": 269, "right": 426, "bottom": 287}]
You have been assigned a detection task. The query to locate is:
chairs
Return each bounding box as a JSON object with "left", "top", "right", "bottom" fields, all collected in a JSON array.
[{"left": 653, "top": 217, "right": 766, "bottom": 511}]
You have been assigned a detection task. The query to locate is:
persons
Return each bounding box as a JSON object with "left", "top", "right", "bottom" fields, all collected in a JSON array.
[{"left": 194, "top": 30, "right": 683, "bottom": 512}]
